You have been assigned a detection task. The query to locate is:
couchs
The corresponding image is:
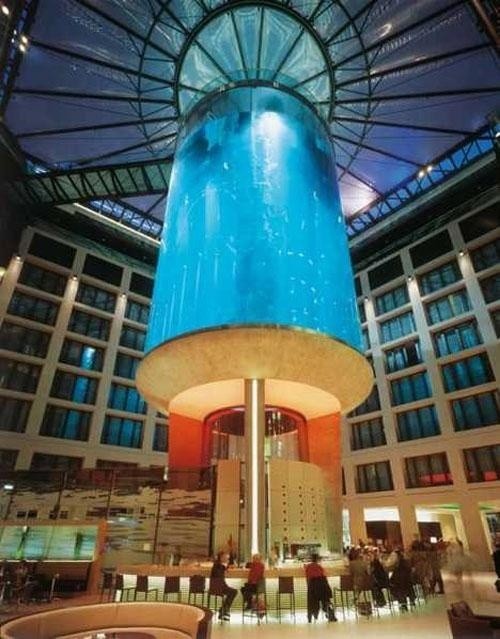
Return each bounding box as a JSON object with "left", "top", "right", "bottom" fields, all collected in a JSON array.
[{"left": 0, "top": 601, "right": 214, "bottom": 639}]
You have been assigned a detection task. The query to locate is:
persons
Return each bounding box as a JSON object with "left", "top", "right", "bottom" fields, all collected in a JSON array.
[
  {"left": 209, "top": 550, "right": 236, "bottom": 621},
  {"left": 488, "top": 532, "right": 499, "bottom": 594},
  {"left": 239, "top": 553, "right": 265, "bottom": 611},
  {"left": 301, "top": 532, "right": 465, "bottom": 623},
  {"left": 1, "top": 558, "right": 61, "bottom": 608}
]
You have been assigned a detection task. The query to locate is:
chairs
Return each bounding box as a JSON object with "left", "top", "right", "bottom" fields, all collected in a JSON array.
[
  {"left": 99, "top": 571, "right": 394, "bottom": 626},
  {"left": 446, "top": 598, "right": 500, "bottom": 639}
]
